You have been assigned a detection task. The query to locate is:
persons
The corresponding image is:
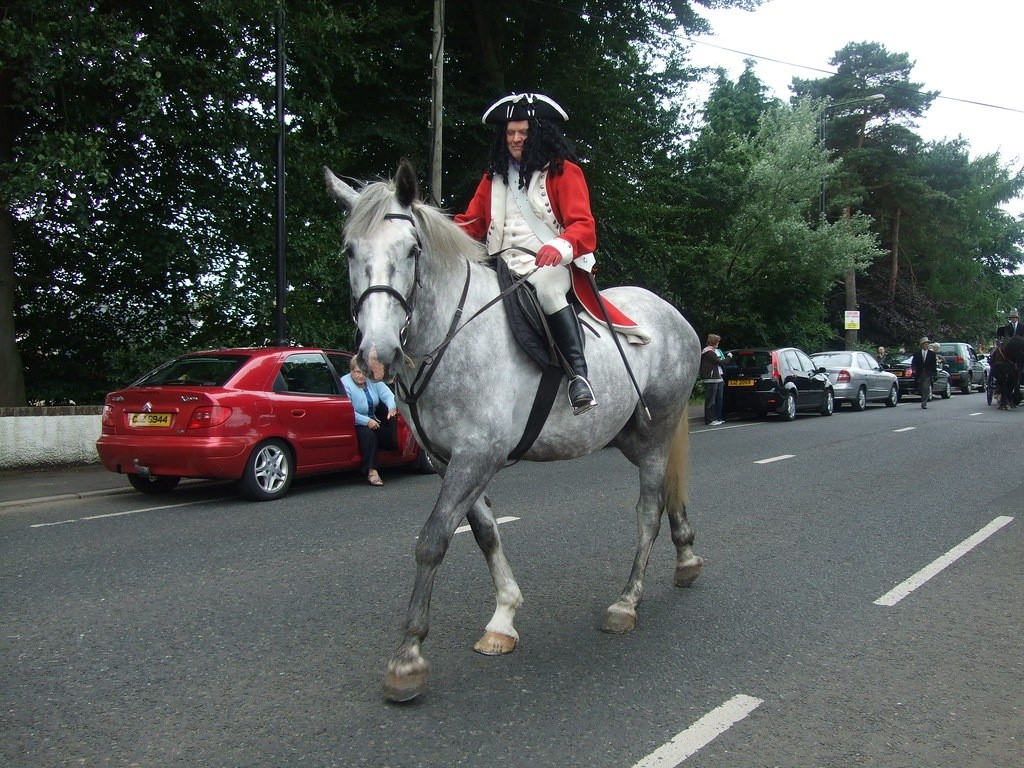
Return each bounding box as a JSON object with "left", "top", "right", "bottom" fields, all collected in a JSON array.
[
  {"left": 700, "top": 334, "right": 733, "bottom": 426},
  {"left": 1006, "top": 311, "right": 1024, "bottom": 336},
  {"left": 340, "top": 354, "right": 398, "bottom": 487},
  {"left": 876, "top": 346, "right": 887, "bottom": 363},
  {"left": 451, "top": 87, "right": 651, "bottom": 417},
  {"left": 912, "top": 337, "right": 936, "bottom": 409},
  {"left": 932, "top": 342, "right": 940, "bottom": 353}
]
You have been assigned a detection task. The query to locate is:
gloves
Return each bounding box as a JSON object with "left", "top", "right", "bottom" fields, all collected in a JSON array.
[
  {"left": 934, "top": 376, "right": 938, "bottom": 382},
  {"left": 912, "top": 371, "right": 916, "bottom": 376},
  {"left": 535, "top": 245, "right": 562, "bottom": 268}
]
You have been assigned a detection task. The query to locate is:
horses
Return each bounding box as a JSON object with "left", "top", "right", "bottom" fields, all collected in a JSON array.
[
  {"left": 990, "top": 335, "right": 1024, "bottom": 410},
  {"left": 322, "top": 157, "right": 702, "bottom": 703}
]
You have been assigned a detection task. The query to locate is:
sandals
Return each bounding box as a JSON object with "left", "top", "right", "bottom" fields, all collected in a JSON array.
[{"left": 367, "top": 474, "right": 384, "bottom": 486}]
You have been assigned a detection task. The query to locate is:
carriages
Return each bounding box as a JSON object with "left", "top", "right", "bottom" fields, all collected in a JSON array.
[{"left": 985, "top": 335, "right": 1024, "bottom": 411}]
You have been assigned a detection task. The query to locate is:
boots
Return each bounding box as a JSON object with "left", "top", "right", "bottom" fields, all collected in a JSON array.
[{"left": 545, "top": 302, "right": 594, "bottom": 406}]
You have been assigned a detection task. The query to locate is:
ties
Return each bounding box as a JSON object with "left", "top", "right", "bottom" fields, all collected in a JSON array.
[{"left": 351, "top": 376, "right": 374, "bottom": 419}]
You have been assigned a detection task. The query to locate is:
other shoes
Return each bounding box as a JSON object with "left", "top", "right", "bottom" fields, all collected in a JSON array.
[{"left": 922, "top": 404, "right": 927, "bottom": 409}]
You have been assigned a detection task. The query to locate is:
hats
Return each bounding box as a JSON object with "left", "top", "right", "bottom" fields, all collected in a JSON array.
[
  {"left": 1009, "top": 311, "right": 1019, "bottom": 318},
  {"left": 919, "top": 337, "right": 931, "bottom": 347},
  {"left": 482, "top": 92, "right": 569, "bottom": 124}
]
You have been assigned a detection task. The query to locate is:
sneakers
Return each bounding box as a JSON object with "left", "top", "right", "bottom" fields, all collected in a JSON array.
[
  {"left": 721, "top": 420, "right": 725, "bottom": 423},
  {"left": 716, "top": 420, "right": 722, "bottom": 425},
  {"left": 708, "top": 421, "right": 720, "bottom": 425}
]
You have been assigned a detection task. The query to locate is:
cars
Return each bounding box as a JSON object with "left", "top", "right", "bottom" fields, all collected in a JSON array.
[
  {"left": 96, "top": 346, "right": 440, "bottom": 502},
  {"left": 722, "top": 346, "right": 834, "bottom": 421},
  {"left": 809, "top": 350, "right": 899, "bottom": 411},
  {"left": 878, "top": 343, "right": 991, "bottom": 398}
]
